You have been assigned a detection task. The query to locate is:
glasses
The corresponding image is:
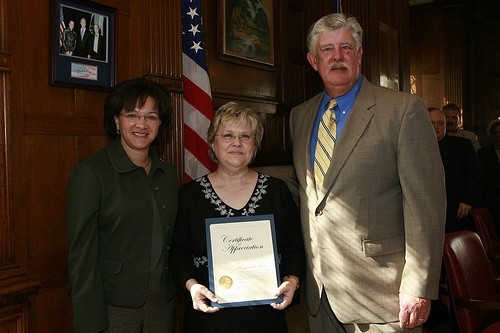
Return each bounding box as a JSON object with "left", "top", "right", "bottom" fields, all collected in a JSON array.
[
  {"left": 117, "top": 111, "right": 161, "bottom": 123},
  {"left": 213, "top": 130, "right": 256, "bottom": 144}
]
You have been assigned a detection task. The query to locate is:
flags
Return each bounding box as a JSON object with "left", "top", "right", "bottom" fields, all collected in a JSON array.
[
  {"left": 58, "top": 8, "right": 67, "bottom": 48},
  {"left": 182, "top": 0, "right": 218, "bottom": 183}
]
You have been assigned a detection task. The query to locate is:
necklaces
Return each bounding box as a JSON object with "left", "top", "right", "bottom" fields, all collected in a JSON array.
[{"left": 132, "top": 158, "right": 150, "bottom": 168}]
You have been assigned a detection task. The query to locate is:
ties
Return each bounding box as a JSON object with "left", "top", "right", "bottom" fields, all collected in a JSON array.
[{"left": 313, "top": 99, "right": 337, "bottom": 200}]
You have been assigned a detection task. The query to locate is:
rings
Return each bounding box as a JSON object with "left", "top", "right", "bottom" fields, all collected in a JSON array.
[{"left": 419, "top": 318, "right": 427, "bottom": 322}]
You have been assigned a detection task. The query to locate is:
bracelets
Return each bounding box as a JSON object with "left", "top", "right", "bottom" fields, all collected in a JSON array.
[{"left": 283, "top": 276, "right": 302, "bottom": 288}]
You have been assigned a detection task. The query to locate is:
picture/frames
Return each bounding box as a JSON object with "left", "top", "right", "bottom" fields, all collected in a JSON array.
[
  {"left": 48, "top": 0, "right": 119, "bottom": 93},
  {"left": 212, "top": 0, "right": 277, "bottom": 73}
]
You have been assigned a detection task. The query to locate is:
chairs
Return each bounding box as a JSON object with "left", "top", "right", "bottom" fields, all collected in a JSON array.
[{"left": 443, "top": 208, "right": 500, "bottom": 333}]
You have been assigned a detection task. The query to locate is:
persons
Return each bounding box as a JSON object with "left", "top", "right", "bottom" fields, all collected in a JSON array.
[
  {"left": 443, "top": 103, "right": 485, "bottom": 209},
  {"left": 426, "top": 107, "right": 477, "bottom": 286},
  {"left": 92, "top": 25, "right": 106, "bottom": 60},
  {"left": 76, "top": 17, "right": 93, "bottom": 58},
  {"left": 487, "top": 117, "right": 500, "bottom": 179},
  {"left": 67, "top": 77, "right": 179, "bottom": 333},
  {"left": 291, "top": 14, "right": 446, "bottom": 332},
  {"left": 170, "top": 103, "right": 305, "bottom": 333},
  {"left": 62, "top": 20, "right": 78, "bottom": 56}
]
